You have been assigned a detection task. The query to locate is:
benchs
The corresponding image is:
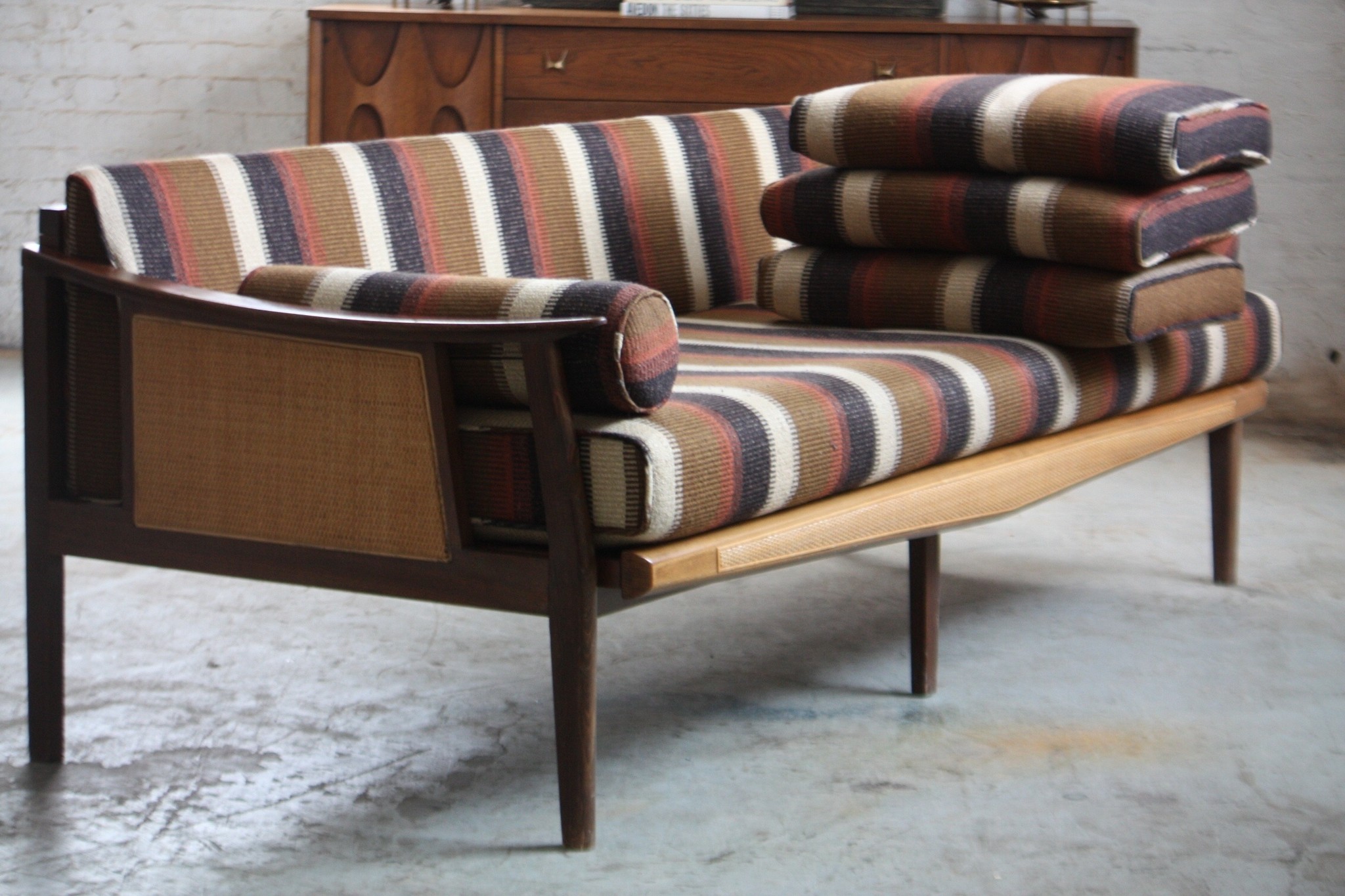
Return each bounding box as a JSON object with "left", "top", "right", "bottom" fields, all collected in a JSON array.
[{"left": 21, "top": 103, "right": 1280, "bottom": 850}]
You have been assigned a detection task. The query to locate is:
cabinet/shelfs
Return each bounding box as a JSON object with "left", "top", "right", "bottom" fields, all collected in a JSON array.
[{"left": 307, "top": 7, "right": 1141, "bottom": 145}]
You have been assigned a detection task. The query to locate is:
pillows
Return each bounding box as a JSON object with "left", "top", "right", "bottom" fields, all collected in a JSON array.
[
  {"left": 755, "top": 246, "right": 1246, "bottom": 347},
  {"left": 237, "top": 264, "right": 680, "bottom": 417},
  {"left": 760, "top": 167, "right": 1258, "bottom": 273},
  {"left": 788, "top": 74, "right": 1272, "bottom": 186}
]
